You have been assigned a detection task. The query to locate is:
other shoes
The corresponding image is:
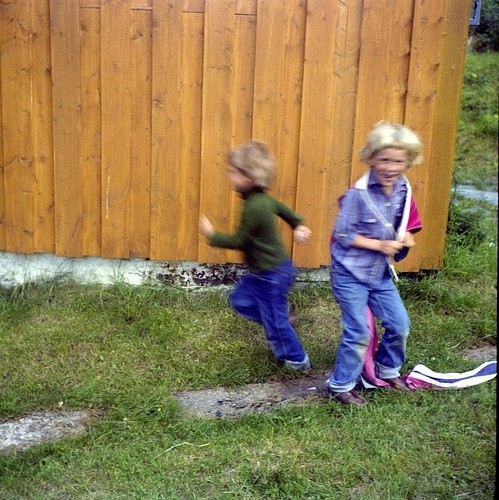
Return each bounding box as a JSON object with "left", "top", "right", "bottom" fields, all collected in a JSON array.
[
  {"left": 383, "top": 375, "right": 410, "bottom": 392},
  {"left": 324, "top": 386, "right": 367, "bottom": 407}
]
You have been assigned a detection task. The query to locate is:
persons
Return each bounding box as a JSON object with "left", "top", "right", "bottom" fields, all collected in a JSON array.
[
  {"left": 197, "top": 141, "right": 312, "bottom": 373},
  {"left": 324, "top": 121, "right": 422, "bottom": 406}
]
